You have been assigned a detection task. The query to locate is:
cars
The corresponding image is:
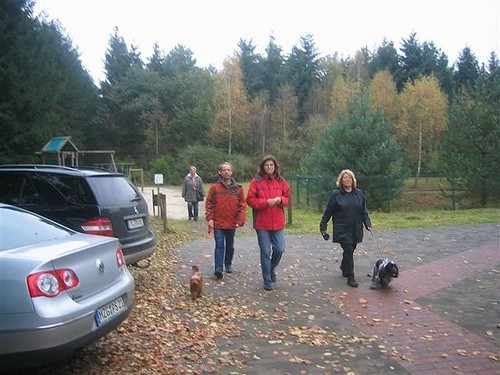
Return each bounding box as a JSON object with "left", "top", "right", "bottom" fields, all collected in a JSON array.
[{"left": 0, "top": 201, "right": 136, "bottom": 372}]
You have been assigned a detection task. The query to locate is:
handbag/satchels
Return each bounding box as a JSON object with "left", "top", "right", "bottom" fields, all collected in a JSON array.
[{"left": 197, "top": 192, "right": 203, "bottom": 201}]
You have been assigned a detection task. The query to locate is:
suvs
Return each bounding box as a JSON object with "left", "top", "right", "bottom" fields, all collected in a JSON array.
[{"left": 0, "top": 162, "right": 159, "bottom": 266}]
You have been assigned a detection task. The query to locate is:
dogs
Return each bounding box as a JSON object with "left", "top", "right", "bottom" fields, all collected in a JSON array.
[
  {"left": 366, "top": 258, "right": 398, "bottom": 290},
  {"left": 190, "top": 265, "right": 203, "bottom": 299}
]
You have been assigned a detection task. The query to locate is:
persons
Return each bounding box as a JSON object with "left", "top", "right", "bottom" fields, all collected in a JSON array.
[
  {"left": 319, "top": 169, "right": 372, "bottom": 288},
  {"left": 205, "top": 162, "right": 246, "bottom": 278},
  {"left": 246, "top": 154, "right": 290, "bottom": 291},
  {"left": 182, "top": 166, "right": 205, "bottom": 221}
]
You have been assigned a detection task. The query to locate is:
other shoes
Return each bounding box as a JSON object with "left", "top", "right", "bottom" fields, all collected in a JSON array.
[
  {"left": 348, "top": 277, "right": 358, "bottom": 287},
  {"left": 194, "top": 217, "right": 198, "bottom": 220},
  {"left": 214, "top": 271, "right": 223, "bottom": 278},
  {"left": 265, "top": 284, "right": 274, "bottom": 291},
  {"left": 343, "top": 272, "right": 349, "bottom": 277},
  {"left": 272, "top": 272, "right": 277, "bottom": 281},
  {"left": 224, "top": 267, "right": 233, "bottom": 273},
  {"left": 189, "top": 214, "right": 193, "bottom": 220}
]
